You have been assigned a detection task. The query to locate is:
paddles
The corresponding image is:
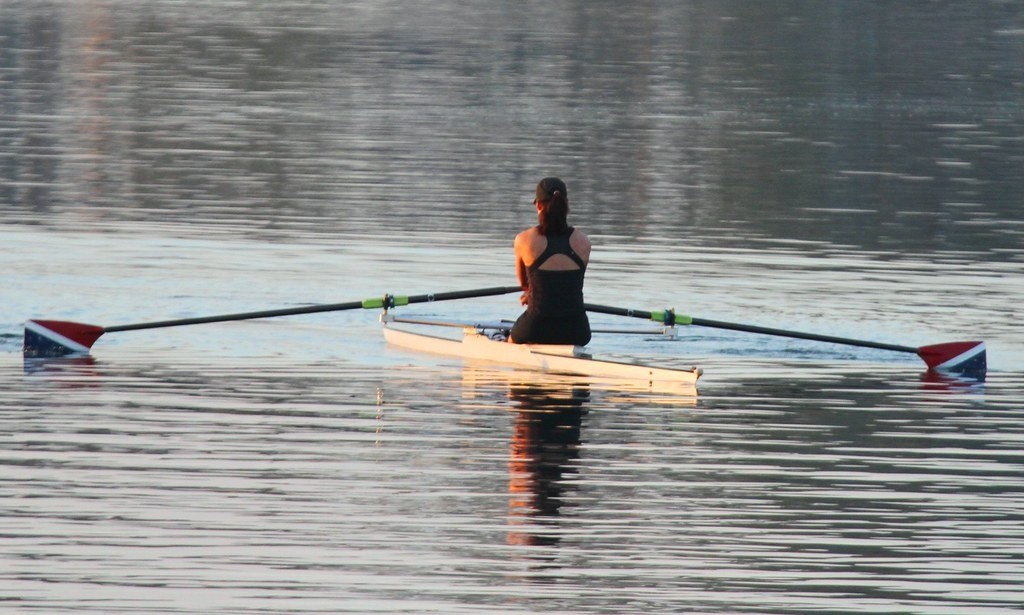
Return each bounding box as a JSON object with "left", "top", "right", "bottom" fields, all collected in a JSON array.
[
  {"left": 583, "top": 302, "right": 987, "bottom": 370},
  {"left": 21, "top": 285, "right": 524, "bottom": 360}
]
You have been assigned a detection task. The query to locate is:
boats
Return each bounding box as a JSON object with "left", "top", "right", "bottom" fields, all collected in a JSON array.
[{"left": 381, "top": 318, "right": 704, "bottom": 384}]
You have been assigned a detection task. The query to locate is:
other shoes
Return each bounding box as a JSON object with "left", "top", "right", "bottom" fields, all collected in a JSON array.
[
  {"left": 503, "top": 328, "right": 513, "bottom": 338},
  {"left": 491, "top": 333, "right": 507, "bottom": 342}
]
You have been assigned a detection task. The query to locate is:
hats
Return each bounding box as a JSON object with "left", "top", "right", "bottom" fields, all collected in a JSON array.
[{"left": 533, "top": 177, "right": 567, "bottom": 204}]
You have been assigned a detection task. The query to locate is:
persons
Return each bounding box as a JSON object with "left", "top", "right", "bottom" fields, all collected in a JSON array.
[{"left": 507, "top": 176, "right": 592, "bottom": 346}]
